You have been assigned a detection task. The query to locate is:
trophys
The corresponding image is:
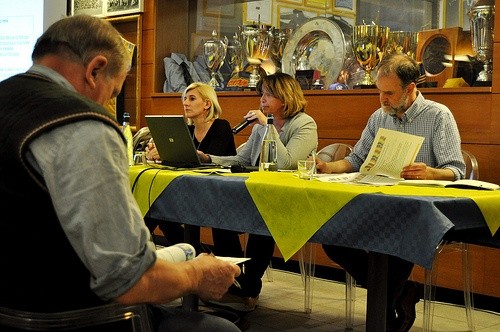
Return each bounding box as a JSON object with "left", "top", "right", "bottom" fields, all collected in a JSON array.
[{"left": 203, "top": 0, "right": 494, "bottom": 89}]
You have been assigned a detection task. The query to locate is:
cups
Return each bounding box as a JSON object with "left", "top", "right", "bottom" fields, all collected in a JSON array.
[
  {"left": 298, "top": 161, "right": 316, "bottom": 180},
  {"left": 134, "top": 151, "right": 147, "bottom": 165}
]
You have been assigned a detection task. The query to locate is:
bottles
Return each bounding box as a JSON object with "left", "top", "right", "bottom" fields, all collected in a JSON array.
[
  {"left": 121, "top": 112, "right": 134, "bottom": 167},
  {"left": 259, "top": 113, "right": 278, "bottom": 171}
]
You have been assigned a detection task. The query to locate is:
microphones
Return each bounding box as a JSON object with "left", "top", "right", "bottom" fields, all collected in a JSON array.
[
  {"left": 232, "top": 117, "right": 257, "bottom": 134},
  {"left": 231, "top": 165, "right": 259, "bottom": 173}
]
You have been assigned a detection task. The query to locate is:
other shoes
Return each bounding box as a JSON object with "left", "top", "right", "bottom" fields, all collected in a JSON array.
[
  {"left": 395, "top": 280, "right": 420, "bottom": 331},
  {"left": 199, "top": 287, "right": 260, "bottom": 310}
]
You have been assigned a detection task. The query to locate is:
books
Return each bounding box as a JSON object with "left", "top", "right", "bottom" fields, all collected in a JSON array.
[
  {"left": 154, "top": 242, "right": 250, "bottom": 305},
  {"left": 400, "top": 179, "right": 500, "bottom": 191}
]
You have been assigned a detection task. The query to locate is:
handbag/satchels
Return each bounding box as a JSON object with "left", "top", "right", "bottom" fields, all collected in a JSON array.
[{"left": 133, "top": 126, "right": 152, "bottom": 153}]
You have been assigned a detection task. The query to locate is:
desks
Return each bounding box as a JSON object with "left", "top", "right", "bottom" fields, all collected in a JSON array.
[{"left": 126, "top": 162, "right": 500, "bottom": 332}]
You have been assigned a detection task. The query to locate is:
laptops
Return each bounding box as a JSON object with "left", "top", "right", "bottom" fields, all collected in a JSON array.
[{"left": 145, "top": 114, "right": 232, "bottom": 169}]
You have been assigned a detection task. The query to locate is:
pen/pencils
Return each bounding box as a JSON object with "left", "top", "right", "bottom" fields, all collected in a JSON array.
[
  {"left": 312, "top": 151, "right": 317, "bottom": 174},
  {"left": 198, "top": 241, "right": 243, "bottom": 292}
]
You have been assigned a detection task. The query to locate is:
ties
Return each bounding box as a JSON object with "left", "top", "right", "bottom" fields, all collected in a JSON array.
[{"left": 181, "top": 61, "right": 191, "bottom": 86}]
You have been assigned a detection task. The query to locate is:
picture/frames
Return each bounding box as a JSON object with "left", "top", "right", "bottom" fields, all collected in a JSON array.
[
  {"left": 189, "top": 0, "right": 357, "bottom": 63},
  {"left": 70, "top": 0, "right": 144, "bottom": 19}
]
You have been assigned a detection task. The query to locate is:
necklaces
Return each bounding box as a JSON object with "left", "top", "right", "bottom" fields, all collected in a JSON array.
[{"left": 191, "top": 121, "right": 212, "bottom": 149}]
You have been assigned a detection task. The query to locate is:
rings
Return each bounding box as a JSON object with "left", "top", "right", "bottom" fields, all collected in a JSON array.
[{"left": 416, "top": 176, "right": 418, "bottom": 179}]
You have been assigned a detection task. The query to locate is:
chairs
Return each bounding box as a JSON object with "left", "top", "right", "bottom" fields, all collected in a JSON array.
[
  {"left": 0, "top": 302, "right": 153, "bottom": 332},
  {"left": 302, "top": 143, "right": 358, "bottom": 332},
  {"left": 405, "top": 149, "right": 481, "bottom": 332}
]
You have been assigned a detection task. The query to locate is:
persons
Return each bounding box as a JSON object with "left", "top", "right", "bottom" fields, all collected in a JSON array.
[
  {"left": 301, "top": 55, "right": 467, "bottom": 331},
  {"left": 198, "top": 72, "right": 319, "bottom": 311},
  {"left": 0, "top": 16, "right": 243, "bottom": 331}
]
